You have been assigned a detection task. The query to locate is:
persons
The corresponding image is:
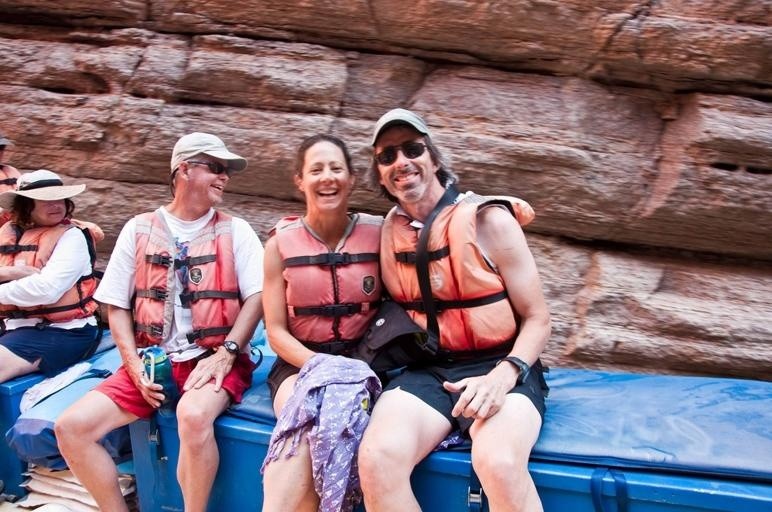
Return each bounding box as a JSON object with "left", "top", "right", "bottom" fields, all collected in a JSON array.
[
  {"left": 51, "top": 131, "right": 265, "bottom": 512},
  {"left": 355, "top": 104, "right": 554, "bottom": 510},
  {"left": 0, "top": 166, "right": 104, "bottom": 388},
  {"left": 1, "top": 132, "right": 22, "bottom": 227},
  {"left": 258, "top": 134, "right": 386, "bottom": 511}
]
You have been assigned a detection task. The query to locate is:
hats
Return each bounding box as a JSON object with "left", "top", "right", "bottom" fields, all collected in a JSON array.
[
  {"left": 0, "top": 168, "right": 89, "bottom": 211},
  {"left": 0, "top": 130, "right": 16, "bottom": 149},
  {"left": 369, "top": 106, "right": 430, "bottom": 147},
  {"left": 168, "top": 131, "right": 250, "bottom": 176}
]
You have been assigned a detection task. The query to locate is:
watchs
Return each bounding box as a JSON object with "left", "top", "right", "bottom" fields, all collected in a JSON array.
[
  {"left": 221, "top": 340, "right": 240, "bottom": 355},
  {"left": 496, "top": 356, "right": 532, "bottom": 385}
]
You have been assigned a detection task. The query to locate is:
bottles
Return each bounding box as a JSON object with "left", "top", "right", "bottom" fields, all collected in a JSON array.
[{"left": 144, "top": 347, "right": 181, "bottom": 420}]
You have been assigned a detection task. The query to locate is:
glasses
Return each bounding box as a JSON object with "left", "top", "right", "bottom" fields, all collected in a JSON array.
[
  {"left": 371, "top": 140, "right": 427, "bottom": 166},
  {"left": 187, "top": 157, "right": 235, "bottom": 180}
]
